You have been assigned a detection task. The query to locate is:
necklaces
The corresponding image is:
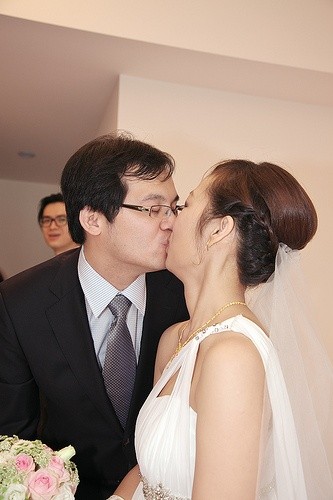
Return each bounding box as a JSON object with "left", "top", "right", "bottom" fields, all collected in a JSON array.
[{"left": 173, "top": 302, "right": 247, "bottom": 353}]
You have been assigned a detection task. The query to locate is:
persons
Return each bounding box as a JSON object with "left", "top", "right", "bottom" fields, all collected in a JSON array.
[
  {"left": 106, "top": 159, "right": 318, "bottom": 500},
  {"left": 0, "top": 129, "right": 191, "bottom": 500},
  {"left": 36, "top": 192, "right": 81, "bottom": 256}
]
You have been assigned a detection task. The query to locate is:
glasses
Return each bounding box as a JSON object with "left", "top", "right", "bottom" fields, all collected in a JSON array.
[
  {"left": 121, "top": 204, "right": 185, "bottom": 220},
  {"left": 40, "top": 215, "right": 67, "bottom": 227}
]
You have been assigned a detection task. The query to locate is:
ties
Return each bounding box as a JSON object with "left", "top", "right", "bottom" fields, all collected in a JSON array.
[{"left": 102, "top": 295, "right": 137, "bottom": 431}]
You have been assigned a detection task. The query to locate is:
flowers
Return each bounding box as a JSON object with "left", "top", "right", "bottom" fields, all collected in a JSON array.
[{"left": 0, "top": 435, "right": 80, "bottom": 500}]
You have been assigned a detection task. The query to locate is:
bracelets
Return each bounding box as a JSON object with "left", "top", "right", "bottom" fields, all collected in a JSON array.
[{"left": 106, "top": 494, "right": 125, "bottom": 500}]
